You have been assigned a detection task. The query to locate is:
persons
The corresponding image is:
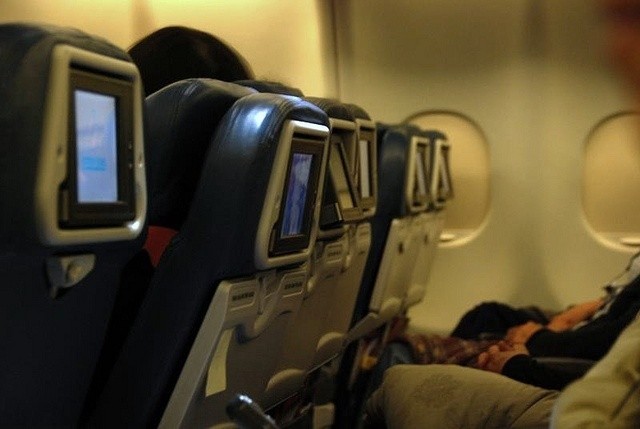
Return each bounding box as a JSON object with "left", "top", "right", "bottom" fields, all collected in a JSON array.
[{"left": 356, "top": 247, "right": 640, "bottom": 428}]
